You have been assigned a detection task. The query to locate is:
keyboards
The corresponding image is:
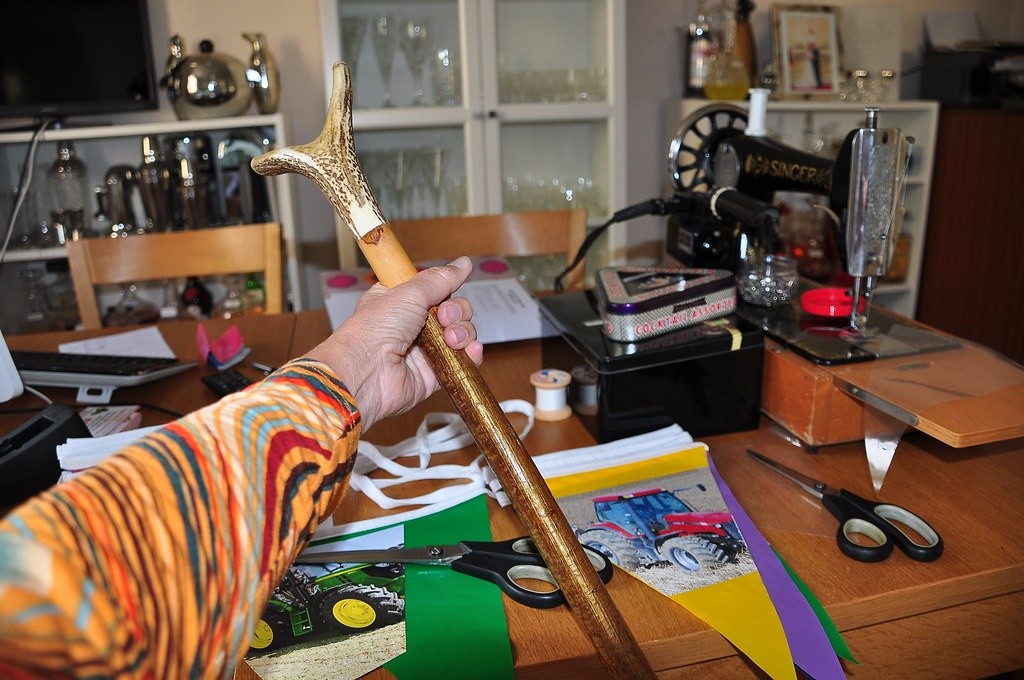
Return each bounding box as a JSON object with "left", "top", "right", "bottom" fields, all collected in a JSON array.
[{"left": 10, "top": 349, "right": 200, "bottom": 404}]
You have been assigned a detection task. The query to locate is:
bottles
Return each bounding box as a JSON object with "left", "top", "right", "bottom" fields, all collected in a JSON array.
[
  {"left": 242, "top": 30, "right": 281, "bottom": 116},
  {"left": 686, "top": 1, "right": 758, "bottom": 102},
  {"left": 0, "top": 143, "right": 88, "bottom": 250},
  {"left": 849, "top": 68, "right": 894, "bottom": 104},
  {"left": 102, "top": 273, "right": 266, "bottom": 329},
  {"left": 41, "top": 258, "right": 79, "bottom": 332}
]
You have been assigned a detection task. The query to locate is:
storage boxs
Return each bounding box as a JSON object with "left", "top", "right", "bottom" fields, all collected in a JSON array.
[
  {"left": 922, "top": 42, "right": 1024, "bottom": 107},
  {"left": 534, "top": 268, "right": 765, "bottom": 457}
]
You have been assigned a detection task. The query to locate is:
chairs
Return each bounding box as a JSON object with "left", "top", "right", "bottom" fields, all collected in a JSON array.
[
  {"left": 386, "top": 208, "right": 594, "bottom": 293},
  {"left": 66, "top": 221, "right": 285, "bottom": 332}
]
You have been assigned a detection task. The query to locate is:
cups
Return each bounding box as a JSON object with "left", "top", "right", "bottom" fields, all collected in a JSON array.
[
  {"left": 433, "top": 47, "right": 463, "bottom": 105},
  {"left": 449, "top": 172, "right": 609, "bottom": 219},
  {"left": 738, "top": 254, "right": 800, "bottom": 307},
  {"left": 496, "top": 49, "right": 608, "bottom": 104},
  {"left": 15, "top": 267, "right": 45, "bottom": 323}
]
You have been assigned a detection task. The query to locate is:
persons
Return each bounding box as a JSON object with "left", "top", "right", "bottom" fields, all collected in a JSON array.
[
  {"left": 793, "top": 42, "right": 825, "bottom": 89},
  {"left": 0, "top": 254, "right": 485, "bottom": 680}
]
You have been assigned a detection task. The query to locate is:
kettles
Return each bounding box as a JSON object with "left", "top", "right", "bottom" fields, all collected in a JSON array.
[
  {"left": 157, "top": 34, "right": 254, "bottom": 121},
  {"left": 137, "top": 127, "right": 277, "bottom": 235},
  {"left": 94, "top": 164, "right": 146, "bottom": 239}
]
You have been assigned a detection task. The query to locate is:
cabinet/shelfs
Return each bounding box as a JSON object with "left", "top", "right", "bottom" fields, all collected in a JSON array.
[
  {"left": 662, "top": 94, "right": 941, "bottom": 322},
  {"left": 914, "top": 106, "right": 1024, "bottom": 367},
  {"left": 0, "top": 110, "right": 299, "bottom": 339},
  {"left": 317, "top": 0, "right": 628, "bottom": 309}
]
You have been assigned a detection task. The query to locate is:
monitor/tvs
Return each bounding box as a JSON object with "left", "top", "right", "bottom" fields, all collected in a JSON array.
[{"left": 0, "top": 0, "right": 159, "bottom": 134}]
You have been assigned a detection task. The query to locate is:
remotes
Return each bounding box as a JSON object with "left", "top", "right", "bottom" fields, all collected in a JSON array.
[{"left": 199, "top": 367, "right": 254, "bottom": 398}]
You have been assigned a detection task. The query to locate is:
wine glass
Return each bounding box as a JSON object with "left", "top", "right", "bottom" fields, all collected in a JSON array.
[
  {"left": 339, "top": 14, "right": 436, "bottom": 107},
  {"left": 357, "top": 146, "right": 447, "bottom": 220}
]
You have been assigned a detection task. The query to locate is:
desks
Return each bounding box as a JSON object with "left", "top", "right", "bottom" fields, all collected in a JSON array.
[{"left": 0, "top": 308, "right": 1024, "bottom": 680}]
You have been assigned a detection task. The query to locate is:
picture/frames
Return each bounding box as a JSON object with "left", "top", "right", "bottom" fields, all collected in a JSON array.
[{"left": 769, "top": 2, "right": 849, "bottom": 102}]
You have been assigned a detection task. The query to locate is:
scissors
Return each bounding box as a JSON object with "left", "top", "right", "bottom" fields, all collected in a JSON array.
[
  {"left": 293, "top": 536, "right": 613, "bottom": 609},
  {"left": 745, "top": 447, "right": 945, "bottom": 564}
]
